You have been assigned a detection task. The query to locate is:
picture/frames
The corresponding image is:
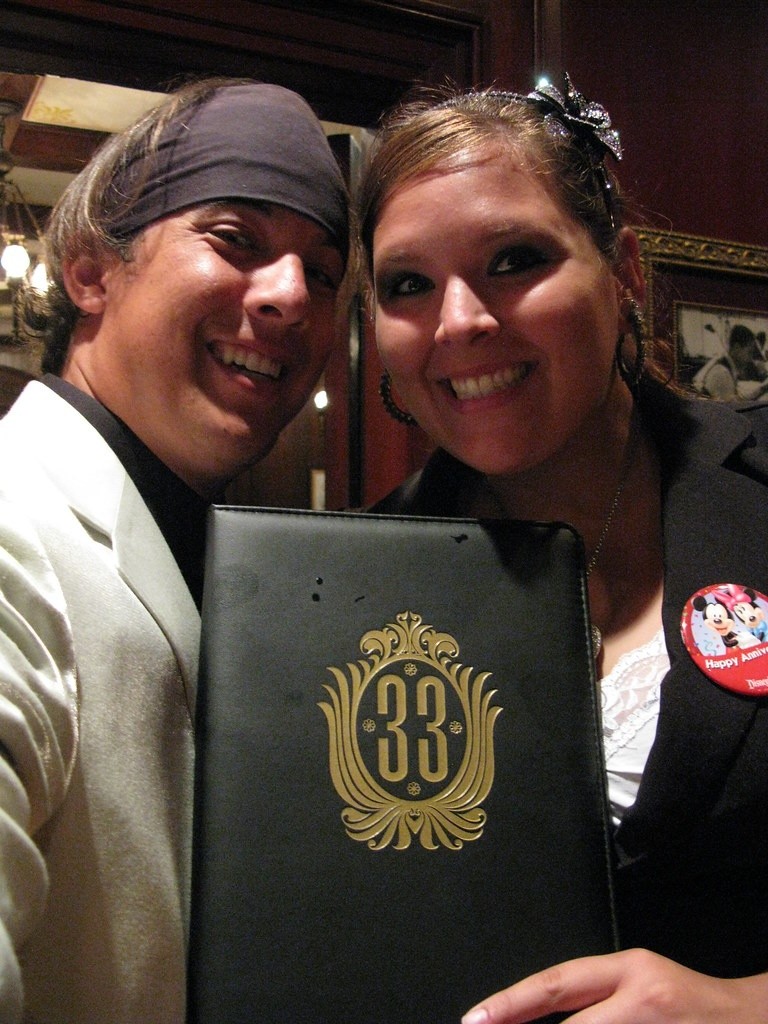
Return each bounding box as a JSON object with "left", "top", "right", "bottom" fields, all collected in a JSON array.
[{"left": 636, "top": 228, "right": 768, "bottom": 407}]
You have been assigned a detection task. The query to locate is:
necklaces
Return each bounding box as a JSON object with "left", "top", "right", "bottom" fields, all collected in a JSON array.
[{"left": 579, "top": 462, "right": 632, "bottom": 658}]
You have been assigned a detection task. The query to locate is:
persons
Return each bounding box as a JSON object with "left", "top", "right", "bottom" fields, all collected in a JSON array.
[
  {"left": 690, "top": 325, "right": 768, "bottom": 403},
  {"left": 348, "top": 77, "right": 768, "bottom": 1024},
  {"left": 1, "top": 80, "right": 374, "bottom": 1024}
]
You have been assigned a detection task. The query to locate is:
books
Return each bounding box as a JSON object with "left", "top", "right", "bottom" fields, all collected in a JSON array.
[{"left": 189, "top": 506, "right": 619, "bottom": 1024}]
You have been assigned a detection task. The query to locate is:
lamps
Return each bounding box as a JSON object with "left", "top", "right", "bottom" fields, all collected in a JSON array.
[{"left": 1, "top": 167, "right": 56, "bottom": 343}]
